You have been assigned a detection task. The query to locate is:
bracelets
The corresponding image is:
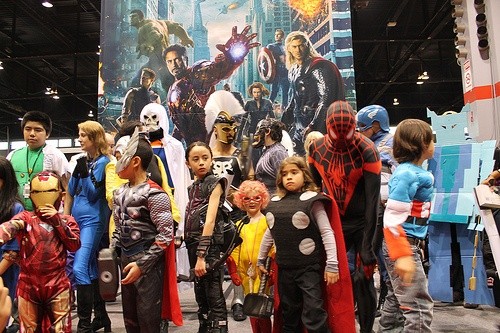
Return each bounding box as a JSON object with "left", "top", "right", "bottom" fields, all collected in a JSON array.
[{"left": 496, "top": 169, "right": 500, "bottom": 174}]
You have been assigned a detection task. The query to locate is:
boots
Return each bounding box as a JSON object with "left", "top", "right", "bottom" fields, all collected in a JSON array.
[
  {"left": 375, "top": 279, "right": 387, "bottom": 317},
  {"left": 75, "top": 279, "right": 112, "bottom": 333}
]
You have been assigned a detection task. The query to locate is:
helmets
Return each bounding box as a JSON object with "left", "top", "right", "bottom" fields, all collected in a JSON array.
[
  {"left": 203, "top": 90, "right": 247, "bottom": 143},
  {"left": 354, "top": 104, "right": 391, "bottom": 133}
]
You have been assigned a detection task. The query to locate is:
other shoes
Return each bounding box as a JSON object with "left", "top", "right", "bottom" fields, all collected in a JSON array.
[
  {"left": 464, "top": 303, "right": 479, "bottom": 308},
  {"left": 231, "top": 303, "right": 248, "bottom": 320},
  {"left": 441, "top": 290, "right": 462, "bottom": 303}
]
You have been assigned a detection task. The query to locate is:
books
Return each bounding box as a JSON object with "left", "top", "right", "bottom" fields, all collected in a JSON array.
[{"left": 474, "top": 184, "right": 500, "bottom": 210}]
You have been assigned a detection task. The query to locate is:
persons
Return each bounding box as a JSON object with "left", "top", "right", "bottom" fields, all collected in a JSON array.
[
  {"left": 115, "top": 8, "right": 345, "bottom": 160},
  {"left": 0, "top": 91, "right": 500, "bottom": 333}
]
